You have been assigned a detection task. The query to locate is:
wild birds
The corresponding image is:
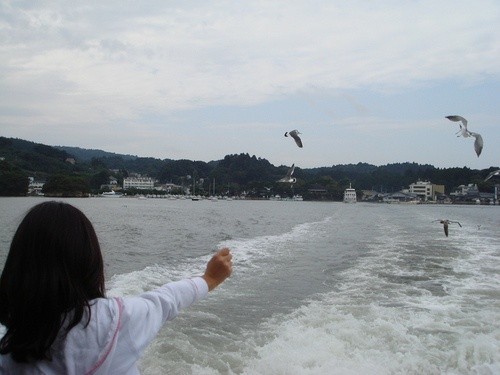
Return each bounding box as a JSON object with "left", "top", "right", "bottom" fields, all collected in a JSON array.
[
  {"left": 274, "top": 163, "right": 296, "bottom": 188},
  {"left": 445, "top": 115, "right": 483, "bottom": 157},
  {"left": 284, "top": 129, "right": 302, "bottom": 148},
  {"left": 432, "top": 219, "right": 462, "bottom": 237}
]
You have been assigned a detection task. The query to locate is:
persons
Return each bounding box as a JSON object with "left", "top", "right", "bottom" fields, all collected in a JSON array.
[{"left": 0, "top": 200, "right": 233, "bottom": 375}]
